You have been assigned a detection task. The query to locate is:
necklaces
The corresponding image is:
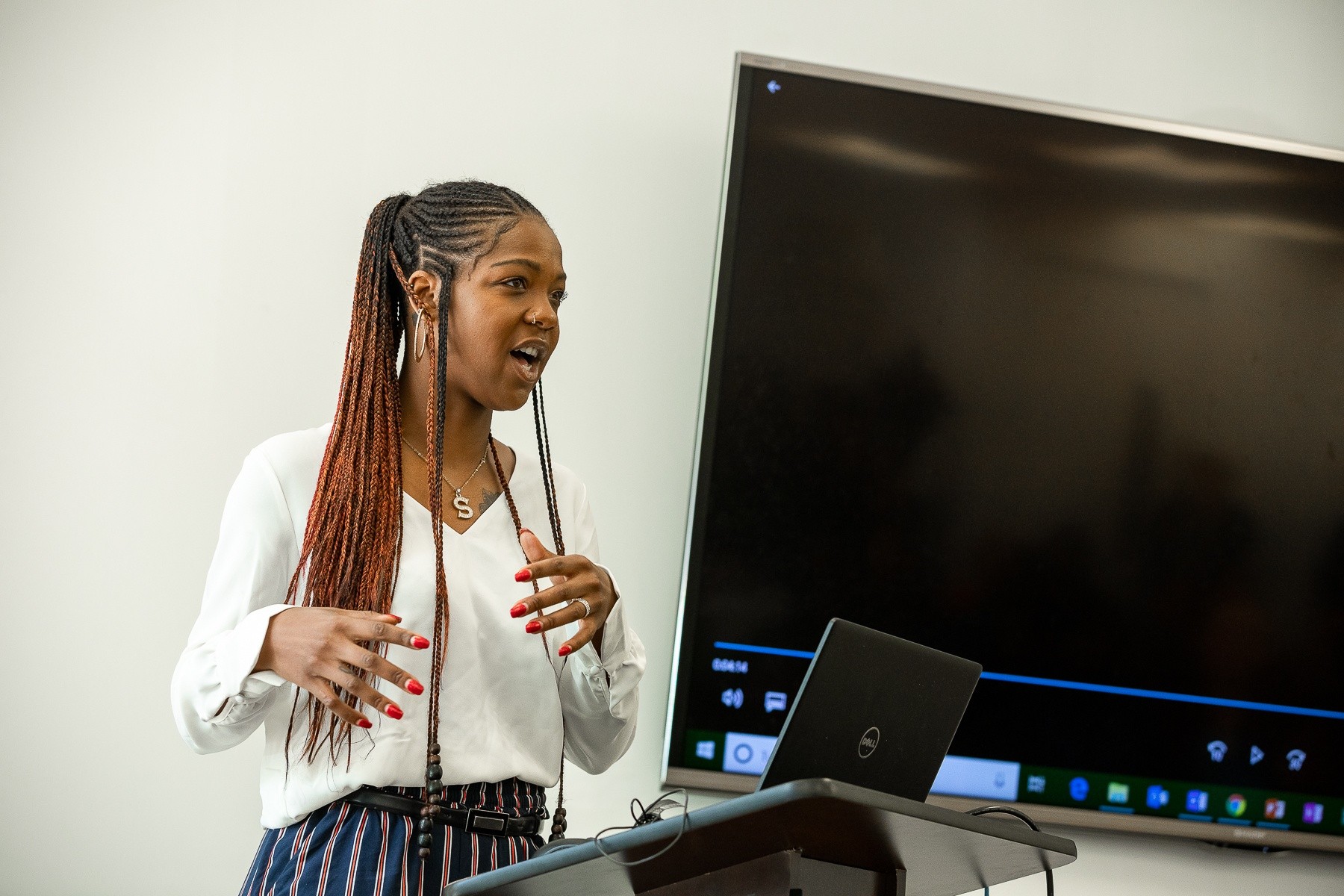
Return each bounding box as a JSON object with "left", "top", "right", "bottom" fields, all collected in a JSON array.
[{"left": 400, "top": 430, "right": 490, "bottom": 520}]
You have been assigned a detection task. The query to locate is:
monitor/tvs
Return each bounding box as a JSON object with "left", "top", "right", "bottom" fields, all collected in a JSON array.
[{"left": 660, "top": 51, "right": 1344, "bottom": 853}]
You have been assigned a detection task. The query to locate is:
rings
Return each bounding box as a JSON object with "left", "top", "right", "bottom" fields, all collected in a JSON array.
[{"left": 569, "top": 596, "right": 592, "bottom": 621}]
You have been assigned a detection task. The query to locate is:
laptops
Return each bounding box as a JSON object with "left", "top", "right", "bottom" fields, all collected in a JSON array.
[{"left": 755, "top": 617, "right": 983, "bottom": 794}]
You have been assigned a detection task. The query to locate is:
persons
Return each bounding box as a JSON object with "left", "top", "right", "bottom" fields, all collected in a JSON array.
[{"left": 173, "top": 181, "right": 647, "bottom": 896}]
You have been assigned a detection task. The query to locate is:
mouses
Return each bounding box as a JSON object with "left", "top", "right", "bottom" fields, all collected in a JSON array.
[{"left": 532, "top": 837, "right": 599, "bottom": 860}]
